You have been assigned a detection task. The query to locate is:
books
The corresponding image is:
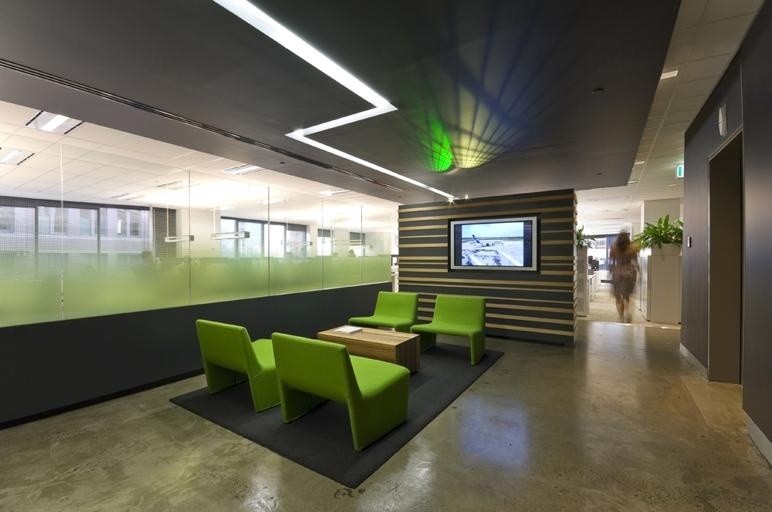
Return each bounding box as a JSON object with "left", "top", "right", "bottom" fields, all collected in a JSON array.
[{"left": 334, "top": 324, "right": 363, "bottom": 335}]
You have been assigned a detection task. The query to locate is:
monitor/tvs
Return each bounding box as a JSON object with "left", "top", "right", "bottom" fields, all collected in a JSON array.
[{"left": 450, "top": 217, "right": 538, "bottom": 272}]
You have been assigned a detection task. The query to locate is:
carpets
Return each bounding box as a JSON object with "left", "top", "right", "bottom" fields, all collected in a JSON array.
[{"left": 168, "top": 343, "right": 505, "bottom": 490}]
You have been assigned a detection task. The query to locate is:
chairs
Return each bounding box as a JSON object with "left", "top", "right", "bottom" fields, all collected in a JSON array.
[
  {"left": 347, "top": 290, "right": 486, "bottom": 366},
  {"left": 194, "top": 317, "right": 411, "bottom": 452}
]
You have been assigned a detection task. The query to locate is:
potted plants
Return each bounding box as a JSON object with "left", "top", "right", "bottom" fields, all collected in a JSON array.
[{"left": 630, "top": 214, "right": 686, "bottom": 257}]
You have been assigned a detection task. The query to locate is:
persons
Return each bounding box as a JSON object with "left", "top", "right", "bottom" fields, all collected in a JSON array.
[
  {"left": 608, "top": 227, "right": 642, "bottom": 324},
  {"left": 140, "top": 249, "right": 154, "bottom": 267}
]
